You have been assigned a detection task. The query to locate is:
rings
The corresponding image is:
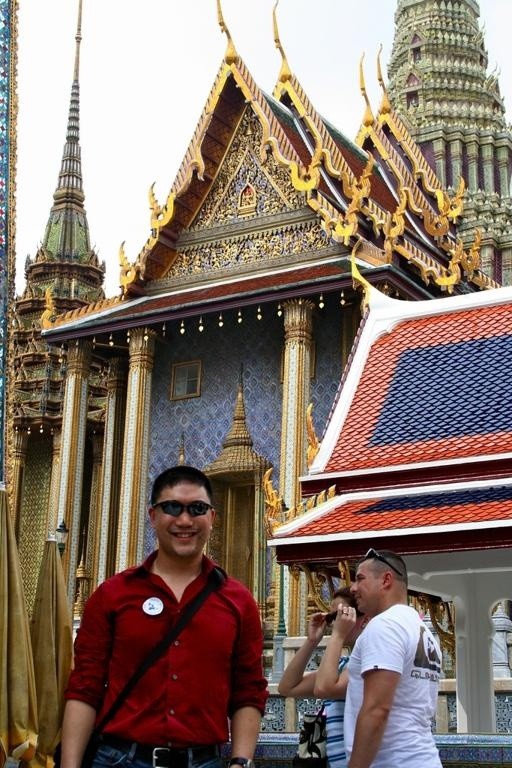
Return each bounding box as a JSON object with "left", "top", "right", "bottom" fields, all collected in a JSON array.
[{"left": 343, "top": 611, "right": 348, "bottom": 616}]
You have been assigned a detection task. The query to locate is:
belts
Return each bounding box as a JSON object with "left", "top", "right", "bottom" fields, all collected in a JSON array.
[{"left": 104, "top": 735, "right": 218, "bottom": 767}]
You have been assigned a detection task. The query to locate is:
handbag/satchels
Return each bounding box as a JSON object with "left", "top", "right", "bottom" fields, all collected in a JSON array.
[
  {"left": 53, "top": 729, "right": 99, "bottom": 768},
  {"left": 291, "top": 713, "right": 327, "bottom": 767}
]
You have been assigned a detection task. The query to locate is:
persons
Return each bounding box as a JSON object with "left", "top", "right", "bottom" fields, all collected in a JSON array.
[
  {"left": 275, "top": 583, "right": 378, "bottom": 768},
  {"left": 48, "top": 463, "right": 270, "bottom": 768},
  {"left": 336, "top": 549, "right": 445, "bottom": 768}
]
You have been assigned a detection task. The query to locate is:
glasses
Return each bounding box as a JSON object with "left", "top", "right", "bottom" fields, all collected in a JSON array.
[
  {"left": 151, "top": 500, "right": 214, "bottom": 516},
  {"left": 365, "top": 547, "right": 403, "bottom": 575},
  {"left": 326, "top": 609, "right": 364, "bottom": 625}
]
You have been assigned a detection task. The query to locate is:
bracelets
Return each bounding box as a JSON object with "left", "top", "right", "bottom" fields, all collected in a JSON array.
[{"left": 230, "top": 756, "right": 256, "bottom": 768}]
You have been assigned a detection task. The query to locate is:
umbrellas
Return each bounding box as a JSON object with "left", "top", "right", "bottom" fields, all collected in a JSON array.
[
  {"left": 21, "top": 528, "right": 76, "bottom": 768},
  {"left": 0, "top": 476, "right": 40, "bottom": 767}
]
